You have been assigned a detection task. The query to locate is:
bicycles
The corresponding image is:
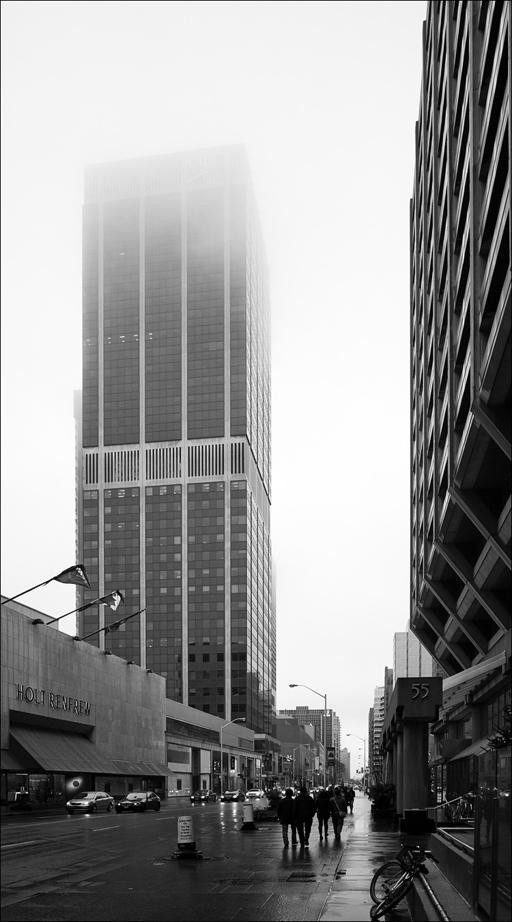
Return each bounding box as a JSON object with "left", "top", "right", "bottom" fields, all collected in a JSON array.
[{"left": 370, "top": 833, "right": 439, "bottom": 918}]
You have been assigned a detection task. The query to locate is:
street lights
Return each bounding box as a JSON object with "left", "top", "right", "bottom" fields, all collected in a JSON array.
[
  {"left": 347, "top": 733, "right": 366, "bottom": 795},
  {"left": 221, "top": 718, "right": 246, "bottom": 799},
  {"left": 289, "top": 684, "right": 326, "bottom": 788},
  {"left": 292, "top": 744, "right": 310, "bottom": 783}
]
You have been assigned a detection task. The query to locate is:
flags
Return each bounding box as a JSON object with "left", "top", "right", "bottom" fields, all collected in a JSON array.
[
  {"left": 102, "top": 608, "right": 148, "bottom": 636},
  {"left": 77, "top": 590, "right": 124, "bottom": 612},
  {"left": 53, "top": 565, "right": 91, "bottom": 590}
]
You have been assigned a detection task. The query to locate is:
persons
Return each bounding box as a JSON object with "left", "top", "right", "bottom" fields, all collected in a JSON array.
[
  {"left": 329, "top": 789, "right": 347, "bottom": 840},
  {"left": 292, "top": 786, "right": 316, "bottom": 845},
  {"left": 315, "top": 791, "right": 330, "bottom": 839},
  {"left": 320, "top": 785, "right": 355, "bottom": 814},
  {"left": 277, "top": 788, "right": 298, "bottom": 848}
]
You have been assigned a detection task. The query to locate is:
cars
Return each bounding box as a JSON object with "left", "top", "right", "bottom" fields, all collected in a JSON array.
[
  {"left": 114, "top": 791, "right": 161, "bottom": 811},
  {"left": 247, "top": 788, "right": 264, "bottom": 798},
  {"left": 66, "top": 791, "right": 116, "bottom": 814},
  {"left": 191, "top": 788, "right": 216, "bottom": 803},
  {"left": 221, "top": 791, "right": 246, "bottom": 802}
]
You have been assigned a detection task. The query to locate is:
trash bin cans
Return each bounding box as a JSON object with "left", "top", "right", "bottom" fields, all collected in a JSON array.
[{"left": 405, "top": 808, "right": 428, "bottom": 844}]
